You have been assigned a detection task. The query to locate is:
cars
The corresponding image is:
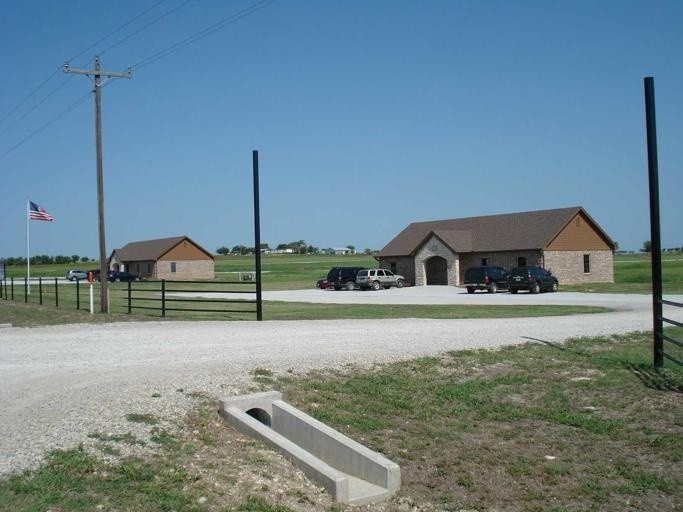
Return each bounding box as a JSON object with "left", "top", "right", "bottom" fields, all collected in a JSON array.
[
  {"left": 506, "top": 267, "right": 558, "bottom": 293},
  {"left": 66, "top": 268, "right": 87, "bottom": 280},
  {"left": 316, "top": 278, "right": 329, "bottom": 289},
  {"left": 463, "top": 266, "right": 510, "bottom": 293},
  {"left": 88, "top": 268, "right": 141, "bottom": 281}
]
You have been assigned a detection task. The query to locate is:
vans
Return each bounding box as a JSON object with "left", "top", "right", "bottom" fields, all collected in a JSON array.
[
  {"left": 326, "top": 266, "right": 368, "bottom": 290},
  {"left": 355, "top": 267, "right": 405, "bottom": 290}
]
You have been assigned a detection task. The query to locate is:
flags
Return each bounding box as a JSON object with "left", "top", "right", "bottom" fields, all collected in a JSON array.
[{"left": 27, "top": 200, "right": 54, "bottom": 221}]
